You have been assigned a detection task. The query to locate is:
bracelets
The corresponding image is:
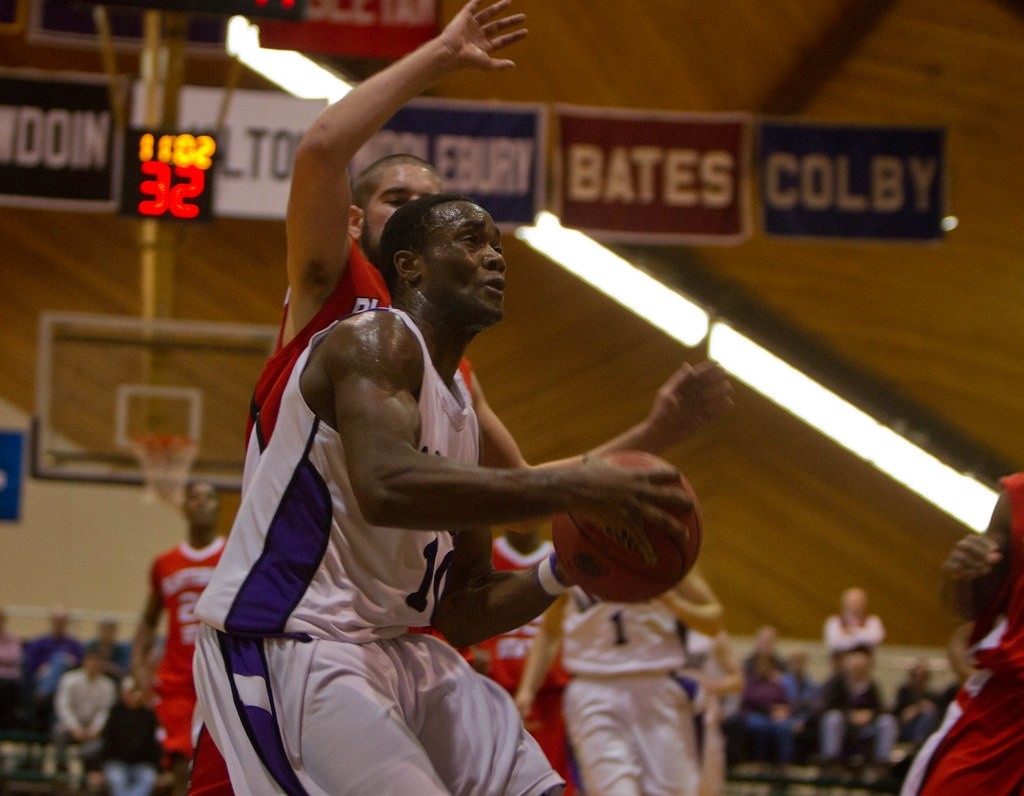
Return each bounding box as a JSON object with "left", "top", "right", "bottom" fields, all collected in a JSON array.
[{"left": 538, "top": 553, "right": 572, "bottom": 596}]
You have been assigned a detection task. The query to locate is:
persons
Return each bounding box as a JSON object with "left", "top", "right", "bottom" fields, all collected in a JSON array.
[
  {"left": 821, "top": 644, "right": 897, "bottom": 779},
  {"left": 823, "top": 588, "right": 886, "bottom": 676},
  {"left": 893, "top": 664, "right": 938, "bottom": 745},
  {"left": 0, "top": 613, "right": 823, "bottom": 796},
  {"left": 132, "top": 481, "right": 230, "bottom": 796},
  {"left": 899, "top": 471, "right": 1024, "bottom": 796},
  {"left": 463, "top": 529, "right": 580, "bottom": 796},
  {"left": 192, "top": 198, "right": 695, "bottom": 796},
  {"left": 517, "top": 571, "right": 724, "bottom": 796},
  {"left": 192, "top": 0, "right": 734, "bottom": 796}
]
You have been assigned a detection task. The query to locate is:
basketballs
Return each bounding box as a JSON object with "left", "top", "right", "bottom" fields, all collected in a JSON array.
[{"left": 543, "top": 444, "right": 709, "bottom": 609}]
